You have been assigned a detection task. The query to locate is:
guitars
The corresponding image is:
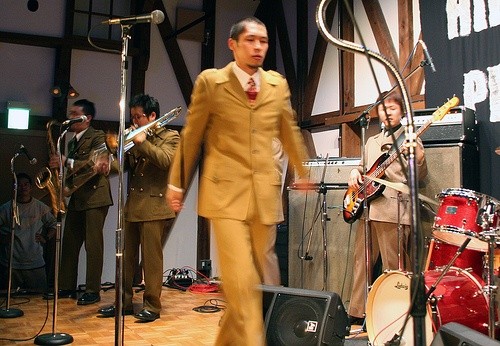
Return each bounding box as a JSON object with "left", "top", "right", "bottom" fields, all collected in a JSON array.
[{"left": 342, "top": 93, "right": 460, "bottom": 224}]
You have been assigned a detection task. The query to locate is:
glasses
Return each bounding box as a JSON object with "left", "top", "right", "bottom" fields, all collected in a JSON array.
[{"left": 130, "top": 113, "right": 147, "bottom": 122}]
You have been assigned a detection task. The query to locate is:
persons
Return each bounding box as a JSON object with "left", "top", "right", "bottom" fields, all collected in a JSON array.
[
  {"left": 0, "top": 173, "right": 56, "bottom": 292},
  {"left": 166, "top": 16, "right": 315, "bottom": 346},
  {"left": 94, "top": 93, "right": 180, "bottom": 321},
  {"left": 347, "top": 91, "right": 429, "bottom": 326},
  {"left": 48, "top": 99, "right": 114, "bottom": 305}
]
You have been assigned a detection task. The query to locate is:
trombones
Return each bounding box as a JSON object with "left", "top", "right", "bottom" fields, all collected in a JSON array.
[{"left": 14, "top": 106, "right": 181, "bottom": 234}]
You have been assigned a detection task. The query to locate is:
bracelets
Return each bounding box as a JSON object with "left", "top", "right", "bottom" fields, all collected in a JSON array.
[{"left": 44, "top": 236, "right": 49, "bottom": 241}]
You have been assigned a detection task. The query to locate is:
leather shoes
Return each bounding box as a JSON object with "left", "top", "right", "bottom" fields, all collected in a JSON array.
[
  {"left": 97, "top": 306, "right": 133, "bottom": 316},
  {"left": 44, "top": 289, "right": 77, "bottom": 299},
  {"left": 135, "top": 310, "right": 160, "bottom": 321},
  {"left": 77, "top": 292, "right": 100, "bottom": 305}
]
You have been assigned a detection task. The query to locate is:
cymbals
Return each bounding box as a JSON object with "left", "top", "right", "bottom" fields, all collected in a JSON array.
[{"left": 362, "top": 174, "right": 440, "bottom": 207}]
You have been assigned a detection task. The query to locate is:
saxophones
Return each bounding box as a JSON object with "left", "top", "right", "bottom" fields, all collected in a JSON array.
[{"left": 34, "top": 119, "right": 67, "bottom": 219}]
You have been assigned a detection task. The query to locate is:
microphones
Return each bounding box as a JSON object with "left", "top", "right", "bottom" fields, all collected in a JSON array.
[
  {"left": 419, "top": 40, "right": 436, "bottom": 73},
  {"left": 102, "top": 9, "right": 165, "bottom": 24},
  {"left": 21, "top": 145, "right": 37, "bottom": 164},
  {"left": 385, "top": 333, "right": 401, "bottom": 346},
  {"left": 62, "top": 115, "right": 87, "bottom": 124},
  {"left": 300, "top": 256, "right": 313, "bottom": 260}
]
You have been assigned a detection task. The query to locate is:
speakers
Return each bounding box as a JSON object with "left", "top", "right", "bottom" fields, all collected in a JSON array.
[
  {"left": 260, "top": 284, "right": 351, "bottom": 346},
  {"left": 430, "top": 322, "right": 500, "bottom": 346},
  {"left": 414, "top": 142, "right": 480, "bottom": 269},
  {"left": 287, "top": 186, "right": 370, "bottom": 319}
]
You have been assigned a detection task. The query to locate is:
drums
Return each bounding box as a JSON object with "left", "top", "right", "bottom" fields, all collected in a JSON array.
[
  {"left": 431, "top": 186, "right": 500, "bottom": 253},
  {"left": 424, "top": 235, "right": 484, "bottom": 279},
  {"left": 364, "top": 265, "right": 491, "bottom": 346}
]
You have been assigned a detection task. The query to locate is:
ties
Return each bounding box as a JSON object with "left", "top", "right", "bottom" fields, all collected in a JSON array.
[{"left": 246, "top": 78, "right": 257, "bottom": 106}]
